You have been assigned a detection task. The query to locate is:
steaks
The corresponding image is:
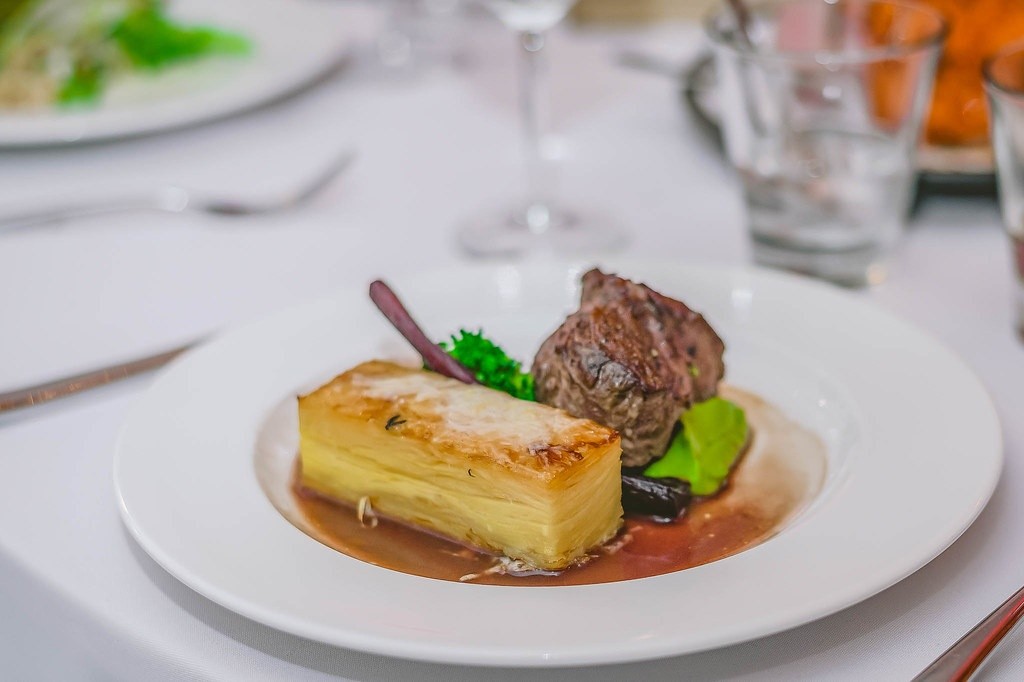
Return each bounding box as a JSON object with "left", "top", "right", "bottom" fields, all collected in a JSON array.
[{"left": 527, "top": 267, "right": 726, "bottom": 467}]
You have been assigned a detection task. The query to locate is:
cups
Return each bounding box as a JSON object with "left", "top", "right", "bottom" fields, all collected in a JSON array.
[
  {"left": 702, "top": 0, "right": 952, "bottom": 286},
  {"left": 980, "top": 44, "right": 1024, "bottom": 344}
]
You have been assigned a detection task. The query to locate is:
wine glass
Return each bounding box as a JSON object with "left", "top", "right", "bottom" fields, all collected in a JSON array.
[{"left": 453, "top": 0, "right": 628, "bottom": 257}]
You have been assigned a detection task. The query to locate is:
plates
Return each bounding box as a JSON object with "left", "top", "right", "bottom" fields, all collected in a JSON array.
[
  {"left": 0, "top": 0, "right": 346, "bottom": 145},
  {"left": 112, "top": 260, "right": 1006, "bottom": 668},
  {"left": 686, "top": 49, "right": 996, "bottom": 191}
]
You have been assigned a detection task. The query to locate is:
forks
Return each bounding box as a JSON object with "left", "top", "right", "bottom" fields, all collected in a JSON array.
[{"left": 0, "top": 148, "right": 353, "bottom": 227}]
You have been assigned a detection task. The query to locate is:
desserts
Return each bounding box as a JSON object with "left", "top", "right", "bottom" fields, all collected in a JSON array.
[{"left": 296, "top": 360, "right": 625, "bottom": 574}]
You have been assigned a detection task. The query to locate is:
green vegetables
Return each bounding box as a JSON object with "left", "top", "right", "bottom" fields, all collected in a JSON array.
[
  {"left": 0, "top": 0, "right": 251, "bottom": 109},
  {"left": 415, "top": 330, "right": 535, "bottom": 402}
]
